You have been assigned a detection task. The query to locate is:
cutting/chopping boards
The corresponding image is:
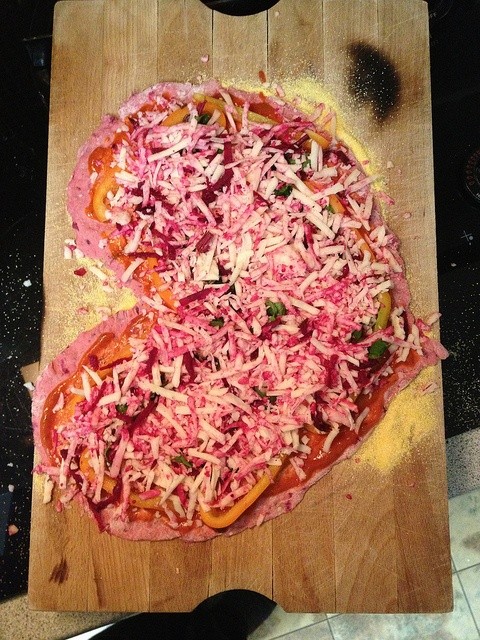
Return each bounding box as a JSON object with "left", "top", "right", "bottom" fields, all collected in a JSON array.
[{"left": 27, "top": 3, "right": 455, "bottom": 616}]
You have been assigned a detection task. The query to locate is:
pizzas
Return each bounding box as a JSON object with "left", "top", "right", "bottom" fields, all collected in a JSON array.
[{"left": 29, "top": 83, "right": 449, "bottom": 543}]
